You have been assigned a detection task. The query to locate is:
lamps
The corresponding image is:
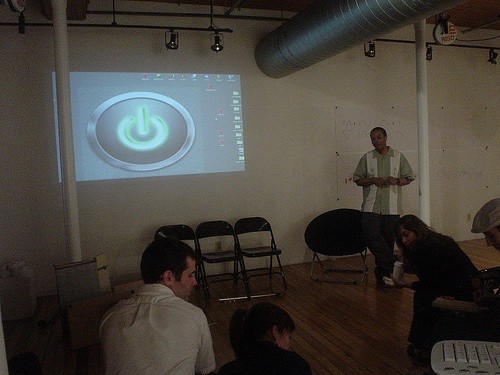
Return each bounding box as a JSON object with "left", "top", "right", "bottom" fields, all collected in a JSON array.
[
  {"left": 364, "top": 40, "right": 375, "bottom": 58},
  {"left": 426, "top": 44, "right": 432, "bottom": 60},
  {"left": 487, "top": 49, "right": 498, "bottom": 65},
  {"left": 165, "top": 30, "right": 178, "bottom": 50},
  {"left": 210, "top": 32, "right": 224, "bottom": 53}
]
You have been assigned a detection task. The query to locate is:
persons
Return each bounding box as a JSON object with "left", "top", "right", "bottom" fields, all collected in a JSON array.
[
  {"left": 217, "top": 302, "right": 312, "bottom": 375},
  {"left": 98, "top": 238, "right": 215, "bottom": 375},
  {"left": 390, "top": 214, "right": 483, "bottom": 352},
  {"left": 353, "top": 127, "right": 416, "bottom": 278},
  {"left": 471, "top": 198, "right": 500, "bottom": 251}
]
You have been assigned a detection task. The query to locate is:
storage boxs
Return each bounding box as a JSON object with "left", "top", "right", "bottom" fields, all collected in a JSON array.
[{"left": 53, "top": 252, "right": 144, "bottom": 350}]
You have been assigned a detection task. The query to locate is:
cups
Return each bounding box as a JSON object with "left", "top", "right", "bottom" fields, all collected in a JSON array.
[{"left": 394, "top": 261, "right": 405, "bottom": 279}]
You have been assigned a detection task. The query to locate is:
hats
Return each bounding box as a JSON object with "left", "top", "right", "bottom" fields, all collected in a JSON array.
[{"left": 471, "top": 198, "right": 500, "bottom": 234}]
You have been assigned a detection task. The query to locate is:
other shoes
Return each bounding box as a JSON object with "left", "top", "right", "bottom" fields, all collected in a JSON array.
[{"left": 407, "top": 344, "right": 430, "bottom": 367}]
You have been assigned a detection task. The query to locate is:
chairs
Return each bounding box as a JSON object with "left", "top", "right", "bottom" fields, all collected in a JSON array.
[
  {"left": 195, "top": 220, "right": 252, "bottom": 301},
  {"left": 304, "top": 208, "right": 369, "bottom": 284},
  {"left": 234, "top": 217, "right": 287, "bottom": 301},
  {"left": 154, "top": 224, "right": 209, "bottom": 298}
]
